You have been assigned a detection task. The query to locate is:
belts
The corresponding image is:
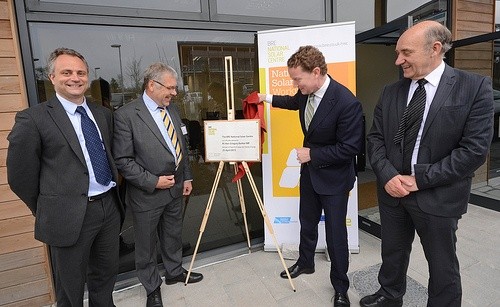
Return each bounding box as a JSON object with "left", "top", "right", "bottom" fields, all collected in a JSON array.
[{"left": 88, "top": 189, "right": 113, "bottom": 202}]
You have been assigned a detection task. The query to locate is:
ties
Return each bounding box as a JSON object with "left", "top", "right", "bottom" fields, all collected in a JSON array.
[
  {"left": 393, "top": 77, "right": 429, "bottom": 175},
  {"left": 158, "top": 106, "right": 183, "bottom": 169},
  {"left": 75, "top": 106, "right": 113, "bottom": 187},
  {"left": 305, "top": 94, "right": 316, "bottom": 131}
]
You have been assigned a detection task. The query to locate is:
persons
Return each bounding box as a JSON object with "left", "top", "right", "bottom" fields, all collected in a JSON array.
[
  {"left": 113, "top": 63, "right": 204, "bottom": 307},
  {"left": 6, "top": 48, "right": 127, "bottom": 307},
  {"left": 360, "top": 21, "right": 495, "bottom": 307},
  {"left": 257, "top": 45, "right": 364, "bottom": 307}
]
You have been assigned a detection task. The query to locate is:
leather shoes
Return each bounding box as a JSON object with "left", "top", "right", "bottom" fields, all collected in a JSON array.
[
  {"left": 147, "top": 285, "right": 163, "bottom": 307},
  {"left": 165, "top": 269, "right": 203, "bottom": 285},
  {"left": 331, "top": 289, "right": 350, "bottom": 307},
  {"left": 281, "top": 263, "right": 315, "bottom": 279},
  {"left": 360, "top": 291, "right": 403, "bottom": 307}
]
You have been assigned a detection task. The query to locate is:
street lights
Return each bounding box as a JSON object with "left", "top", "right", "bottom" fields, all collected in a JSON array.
[
  {"left": 110, "top": 43, "right": 125, "bottom": 105},
  {"left": 94, "top": 67, "right": 100, "bottom": 79}
]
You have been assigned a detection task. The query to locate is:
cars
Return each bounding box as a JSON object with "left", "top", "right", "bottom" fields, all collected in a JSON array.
[{"left": 183, "top": 92, "right": 213, "bottom": 102}]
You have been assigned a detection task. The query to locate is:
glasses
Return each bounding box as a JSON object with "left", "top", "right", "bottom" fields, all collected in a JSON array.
[{"left": 154, "top": 79, "right": 179, "bottom": 92}]
